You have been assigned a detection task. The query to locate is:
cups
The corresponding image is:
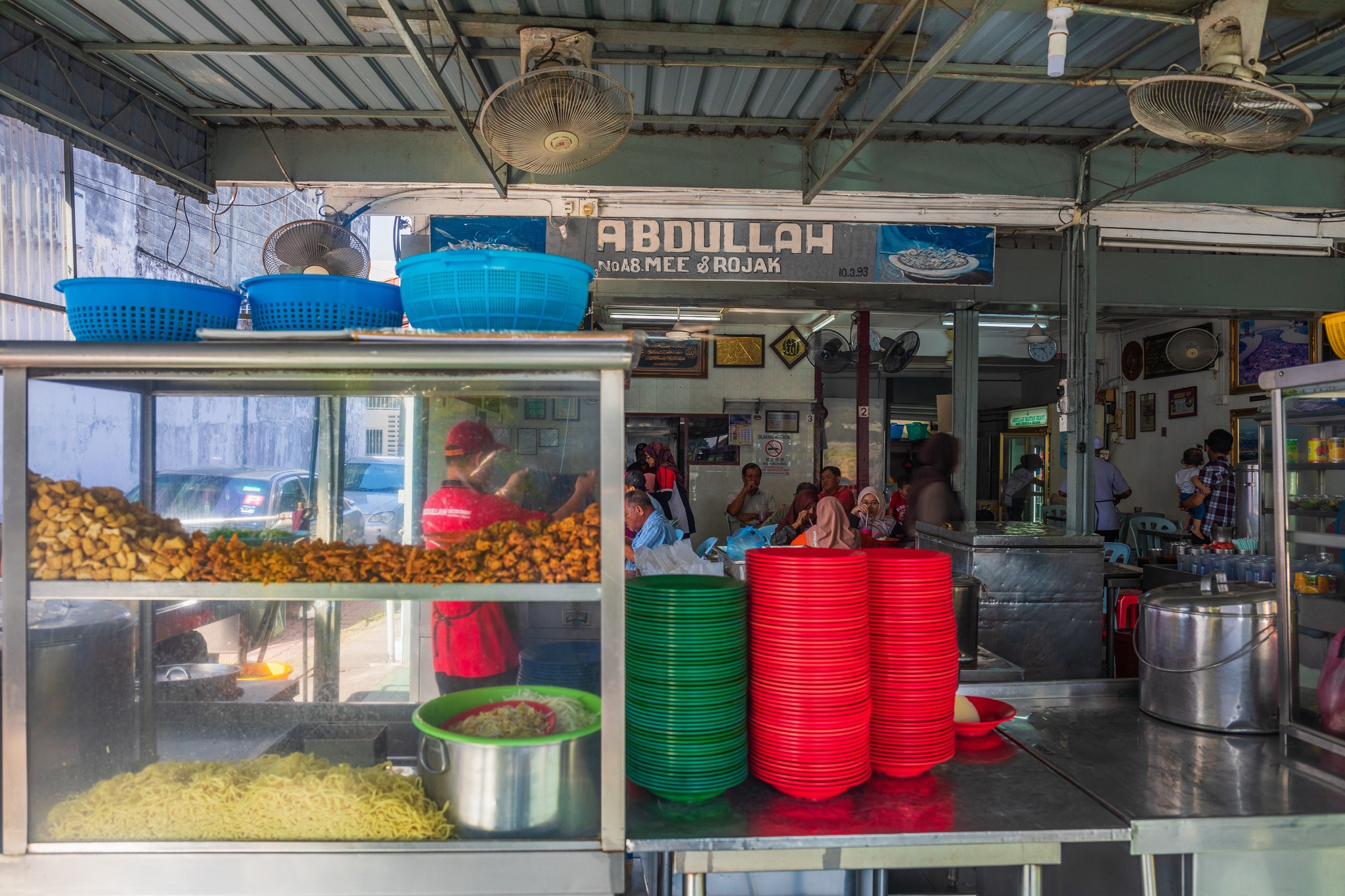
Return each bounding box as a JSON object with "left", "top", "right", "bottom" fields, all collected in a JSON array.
[
  {"left": 861, "top": 525, "right": 872, "bottom": 547},
  {"left": 758, "top": 505, "right": 768, "bottom": 526},
  {"left": 1176, "top": 553, "right": 1345, "bottom": 593},
  {"left": 1148, "top": 548, "right": 1164, "bottom": 564}
]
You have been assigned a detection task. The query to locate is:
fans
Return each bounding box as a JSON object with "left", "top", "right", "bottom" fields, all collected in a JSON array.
[
  {"left": 1128, "top": 0, "right": 1314, "bottom": 149},
  {"left": 806, "top": 329, "right": 859, "bottom": 375},
  {"left": 1166, "top": 328, "right": 1220, "bottom": 381},
  {"left": 869, "top": 331, "right": 920, "bottom": 374},
  {"left": 262, "top": 217, "right": 371, "bottom": 279},
  {"left": 479, "top": 25, "right": 635, "bottom": 174}
]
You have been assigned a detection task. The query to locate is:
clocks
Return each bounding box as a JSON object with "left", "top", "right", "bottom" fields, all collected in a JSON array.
[
  {"left": 1028, "top": 336, "right": 1057, "bottom": 363},
  {"left": 852, "top": 330, "right": 881, "bottom": 352}
]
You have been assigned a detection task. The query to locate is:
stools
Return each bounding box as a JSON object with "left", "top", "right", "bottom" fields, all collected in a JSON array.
[{"left": 1103, "top": 589, "right": 1142, "bottom": 636}]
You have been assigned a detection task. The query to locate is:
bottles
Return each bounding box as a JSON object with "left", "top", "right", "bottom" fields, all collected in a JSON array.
[{"left": 782, "top": 503, "right": 786, "bottom": 507}]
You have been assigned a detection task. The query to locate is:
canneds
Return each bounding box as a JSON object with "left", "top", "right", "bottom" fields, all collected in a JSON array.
[
  {"left": 1286, "top": 439, "right": 1298, "bottom": 463},
  {"left": 1293, "top": 571, "right": 1337, "bottom": 595},
  {"left": 1307, "top": 436, "right": 1345, "bottom": 463}
]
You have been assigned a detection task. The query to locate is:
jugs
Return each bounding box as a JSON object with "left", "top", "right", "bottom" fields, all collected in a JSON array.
[{"left": 1209, "top": 524, "right": 1236, "bottom": 543}]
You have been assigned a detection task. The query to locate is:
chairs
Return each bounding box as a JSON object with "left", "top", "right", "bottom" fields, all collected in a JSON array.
[
  {"left": 670, "top": 517, "right": 778, "bottom": 560},
  {"left": 1040, "top": 503, "right": 1173, "bottom": 629}
]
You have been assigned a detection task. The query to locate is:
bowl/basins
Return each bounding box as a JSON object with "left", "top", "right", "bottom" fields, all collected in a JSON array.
[
  {"left": 874, "top": 538, "right": 900, "bottom": 546},
  {"left": 440, "top": 699, "right": 555, "bottom": 738},
  {"left": 1287, "top": 493, "right": 1345, "bottom": 512},
  {"left": 955, "top": 695, "right": 1017, "bottom": 737},
  {"left": 232, "top": 662, "right": 294, "bottom": 681}
]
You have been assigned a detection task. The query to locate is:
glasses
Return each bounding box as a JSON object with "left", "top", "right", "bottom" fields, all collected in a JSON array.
[
  {"left": 635, "top": 453, "right": 646, "bottom": 457},
  {"left": 861, "top": 502, "right": 879, "bottom": 507},
  {"left": 645, "top": 456, "right": 650, "bottom": 459},
  {"left": 793, "top": 493, "right": 797, "bottom": 497}
]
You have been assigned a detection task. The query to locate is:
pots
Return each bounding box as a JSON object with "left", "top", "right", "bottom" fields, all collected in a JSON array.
[
  {"left": 412, "top": 685, "right": 601, "bottom": 841},
  {"left": 135, "top": 663, "right": 243, "bottom": 702},
  {"left": 1131, "top": 569, "right": 1296, "bottom": 734}
]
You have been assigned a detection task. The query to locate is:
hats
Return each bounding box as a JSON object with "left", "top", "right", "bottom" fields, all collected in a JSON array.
[
  {"left": 624, "top": 470, "right": 647, "bottom": 486},
  {"left": 444, "top": 420, "right": 511, "bottom": 455},
  {"left": 1095, "top": 436, "right": 1103, "bottom": 449},
  {"left": 634, "top": 443, "right": 648, "bottom": 451}
]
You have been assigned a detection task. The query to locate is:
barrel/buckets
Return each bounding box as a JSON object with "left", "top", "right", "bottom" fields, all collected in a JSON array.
[
  {"left": 25, "top": 599, "right": 138, "bottom": 844},
  {"left": 1234, "top": 458, "right": 1259, "bottom": 541}
]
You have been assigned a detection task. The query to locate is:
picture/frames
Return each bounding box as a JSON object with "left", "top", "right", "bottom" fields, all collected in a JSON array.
[
  {"left": 1121, "top": 313, "right": 1319, "bottom": 468},
  {"left": 713, "top": 325, "right": 810, "bottom": 370}
]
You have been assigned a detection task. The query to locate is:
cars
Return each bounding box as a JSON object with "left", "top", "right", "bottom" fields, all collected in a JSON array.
[
  {"left": 343, "top": 454, "right": 407, "bottom": 547},
  {"left": 124, "top": 464, "right": 366, "bottom": 546}
]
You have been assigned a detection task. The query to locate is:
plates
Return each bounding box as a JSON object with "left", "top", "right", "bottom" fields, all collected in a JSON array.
[
  {"left": 516, "top": 640, "right": 601, "bottom": 696},
  {"left": 744, "top": 547, "right": 872, "bottom": 804},
  {"left": 859, "top": 545, "right": 959, "bottom": 779},
  {"left": 626, "top": 573, "right": 748, "bottom": 805}
]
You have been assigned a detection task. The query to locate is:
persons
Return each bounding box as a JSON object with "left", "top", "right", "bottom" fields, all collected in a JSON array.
[
  {"left": 1004, "top": 454, "right": 1050, "bottom": 521},
  {"left": 627, "top": 442, "right": 657, "bottom": 476},
  {"left": 758, "top": 481, "right": 818, "bottom": 542},
  {"left": 910, "top": 432, "right": 965, "bottom": 532},
  {"left": 817, "top": 465, "right": 856, "bottom": 519},
  {"left": 624, "top": 490, "right": 677, "bottom": 571},
  {"left": 887, "top": 474, "right": 917, "bottom": 525},
  {"left": 726, "top": 463, "right": 778, "bottom": 527},
  {"left": 790, "top": 496, "right": 876, "bottom": 549},
  {"left": 643, "top": 441, "right": 696, "bottom": 540},
  {"left": 1175, "top": 428, "right": 1238, "bottom": 546},
  {"left": 1058, "top": 435, "right": 1132, "bottom": 542},
  {"left": 848, "top": 486, "right": 903, "bottom": 539},
  {"left": 624, "top": 470, "right": 666, "bottom": 516},
  {"left": 770, "top": 489, "right": 820, "bottom": 546},
  {"left": 420, "top": 421, "right": 596, "bottom": 698}
]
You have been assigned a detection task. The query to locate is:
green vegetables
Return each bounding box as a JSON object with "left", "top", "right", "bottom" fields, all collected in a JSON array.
[{"left": 1298, "top": 499, "right": 1340, "bottom": 512}]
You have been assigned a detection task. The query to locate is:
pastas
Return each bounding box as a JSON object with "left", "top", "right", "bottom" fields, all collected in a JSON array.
[{"left": 37, "top": 751, "right": 456, "bottom": 841}]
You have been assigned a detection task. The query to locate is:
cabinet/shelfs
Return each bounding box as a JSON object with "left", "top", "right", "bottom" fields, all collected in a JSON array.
[{"left": 0, "top": 328, "right": 645, "bottom": 896}]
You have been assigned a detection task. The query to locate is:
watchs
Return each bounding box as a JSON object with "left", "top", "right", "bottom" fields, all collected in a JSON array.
[{"left": 756, "top": 513, "right": 759, "bottom": 519}]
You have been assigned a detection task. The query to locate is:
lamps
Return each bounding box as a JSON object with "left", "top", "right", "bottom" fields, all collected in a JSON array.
[
  {"left": 1098, "top": 231, "right": 1334, "bottom": 257},
  {"left": 1023, "top": 316, "right": 1049, "bottom": 343},
  {"left": 944, "top": 329, "right": 954, "bottom": 367},
  {"left": 603, "top": 304, "right": 722, "bottom": 321}
]
